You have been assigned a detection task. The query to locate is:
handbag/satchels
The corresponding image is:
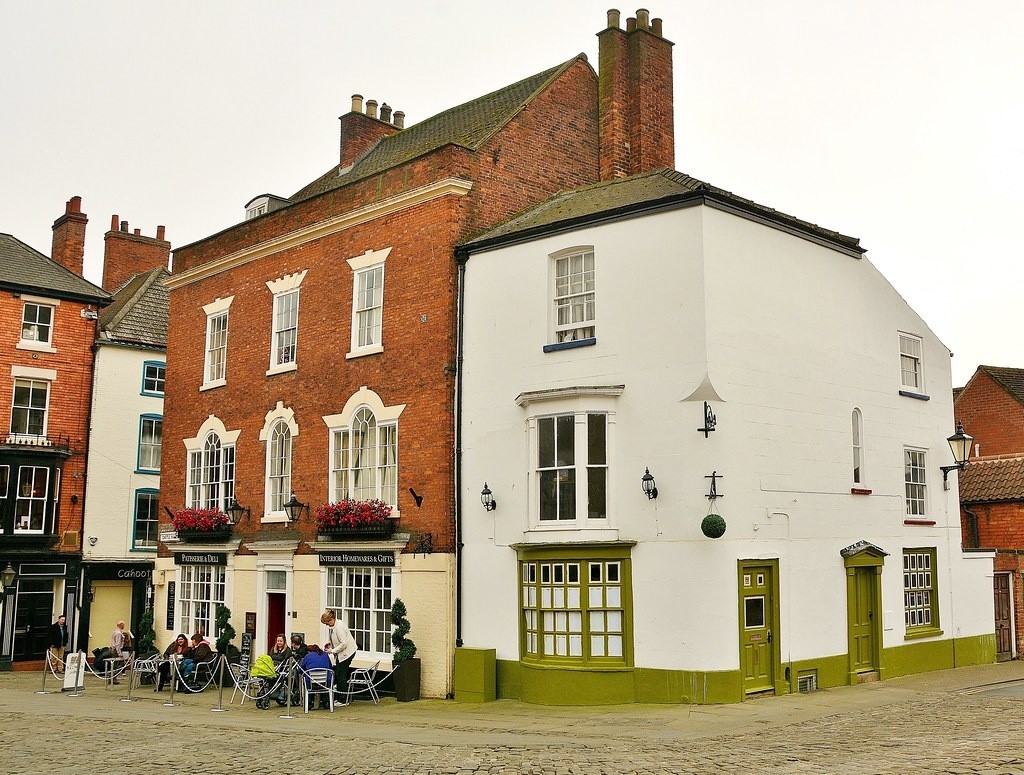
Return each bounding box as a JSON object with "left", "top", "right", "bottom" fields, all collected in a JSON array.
[{"left": 185, "top": 681, "right": 203, "bottom": 693}]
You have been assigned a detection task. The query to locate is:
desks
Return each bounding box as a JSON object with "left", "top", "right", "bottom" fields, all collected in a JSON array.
[{"left": 103, "top": 658, "right": 133, "bottom": 691}]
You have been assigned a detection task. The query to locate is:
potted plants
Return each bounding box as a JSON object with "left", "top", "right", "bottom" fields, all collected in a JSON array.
[
  {"left": 389, "top": 598, "right": 421, "bottom": 702},
  {"left": 215, "top": 605, "right": 240, "bottom": 687},
  {"left": 138, "top": 609, "right": 159, "bottom": 684}
]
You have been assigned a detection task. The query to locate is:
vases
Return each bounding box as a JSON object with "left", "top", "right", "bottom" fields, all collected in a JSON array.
[
  {"left": 317, "top": 523, "right": 395, "bottom": 540},
  {"left": 177, "top": 530, "right": 231, "bottom": 543}
]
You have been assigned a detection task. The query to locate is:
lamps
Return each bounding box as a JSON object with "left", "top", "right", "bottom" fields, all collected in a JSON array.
[
  {"left": 642, "top": 466, "right": 658, "bottom": 500},
  {"left": 479, "top": 482, "right": 497, "bottom": 513},
  {"left": 939, "top": 417, "right": 974, "bottom": 482},
  {"left": 226, "top": 494, "right": 250, "bottom": 524},
  {"left": 283, "top": 489, "right": 310, "bottom": 523}
]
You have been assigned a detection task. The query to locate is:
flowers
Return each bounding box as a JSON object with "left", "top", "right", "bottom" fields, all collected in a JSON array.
[
  {"left": 314, "top": 497, "right": 393, "bottom": 526},
  {"left": 168, "top": 506, "right": 229, "bottom": 532}
]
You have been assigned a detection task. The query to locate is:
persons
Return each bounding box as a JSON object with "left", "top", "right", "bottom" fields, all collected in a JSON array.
[
  {"left": 298, "top": 644, "right": 333, "bottom": 710},
  {"left": 320, "top": 608, "right": 358, "bottom": 706},
  {"left": 49, "top": 614, "right": 69, "bottom": 673},
  {"left": 268, "top": 633, "right": 292, "bottom": 671},
  {"left": 94, "top": 647, "right": 114, "bottom": 676},
  {"left": 108, "top": 620, "right": 125, "bottom": 684},
  {"left": 167, "top": 632, "right": 214, "bottom": 692},
  {"left": 145, "top": 634, "right": 188, "bottom": 691},
  {"left": 289, "top": 635, "right": 309, "bottom": 672}
]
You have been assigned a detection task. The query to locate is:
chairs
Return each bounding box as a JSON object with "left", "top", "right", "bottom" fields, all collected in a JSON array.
[
  {"left": 158, "top": 661, "right": 183, "bottom": 691},
  {"left": 229, "top": 663, "right": 264, "bottom": 705},
  {"left": 300, "top": 668, "right": 335, "bottom": 714},
  {"left": 346, "top": 660, "right": 382, "bottom": 706},
  {"left": 133, "top": 653, "right": 162, "bottom": 688},
  {"left": 191, "top": 653, "right": 218, "bottom": 690}
]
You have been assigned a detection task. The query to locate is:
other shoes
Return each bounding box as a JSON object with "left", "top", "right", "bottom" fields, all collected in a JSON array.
[
  {"left": 59, "top": 671, "right": 64, "bottom": 674},
  {"left": 334, "top": 700, "right": 349, "bottom": 706},
  {"left": 109, "top": 677, "right": 119, "bottom": 684},
  {"left": 154, "top": 686, "right": 162, "bottom": 692},
  {"left": 177, "top": 685, "right": 187, "bottom": 692}
]
